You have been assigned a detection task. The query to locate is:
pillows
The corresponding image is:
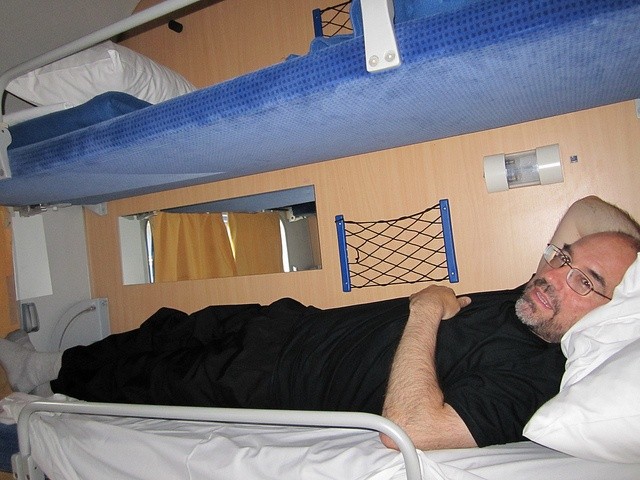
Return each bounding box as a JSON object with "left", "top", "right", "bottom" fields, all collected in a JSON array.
[
  {"left": 520, "top": 334, "right": 638, "bottom": 467},
  {"left": 4, "top": 38, "right": 197, "bottom": 109},
  {"left": 6, "top": 90, "right": 156, "bottom": 151}
]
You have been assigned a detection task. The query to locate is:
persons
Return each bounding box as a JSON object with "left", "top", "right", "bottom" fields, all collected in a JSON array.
[{"left": 1, "top": 195, "right": 640, "bottom": 451}]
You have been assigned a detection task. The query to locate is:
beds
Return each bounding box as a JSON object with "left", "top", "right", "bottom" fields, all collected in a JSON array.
[
  {"left": 0, "top": 252, "right": 639, "bottom": 477},
  {"left": 0, "top": 0, "right": 640, "bottom": 209}
]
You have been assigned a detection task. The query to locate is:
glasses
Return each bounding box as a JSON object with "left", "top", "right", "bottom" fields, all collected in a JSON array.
[{"left": 542, "top": 243, "right": 612, "bottom": 301}]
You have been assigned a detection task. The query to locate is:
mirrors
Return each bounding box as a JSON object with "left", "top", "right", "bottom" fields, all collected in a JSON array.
[{"left": 117, "top": 184, "right": 323, "bottom": 286}]
